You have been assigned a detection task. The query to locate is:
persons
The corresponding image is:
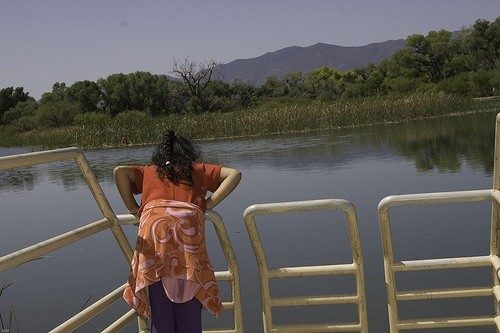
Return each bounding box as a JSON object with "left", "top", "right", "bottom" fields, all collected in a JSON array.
[{"left": 113, "top": 130, "right": 243, "bottom": 333}]
